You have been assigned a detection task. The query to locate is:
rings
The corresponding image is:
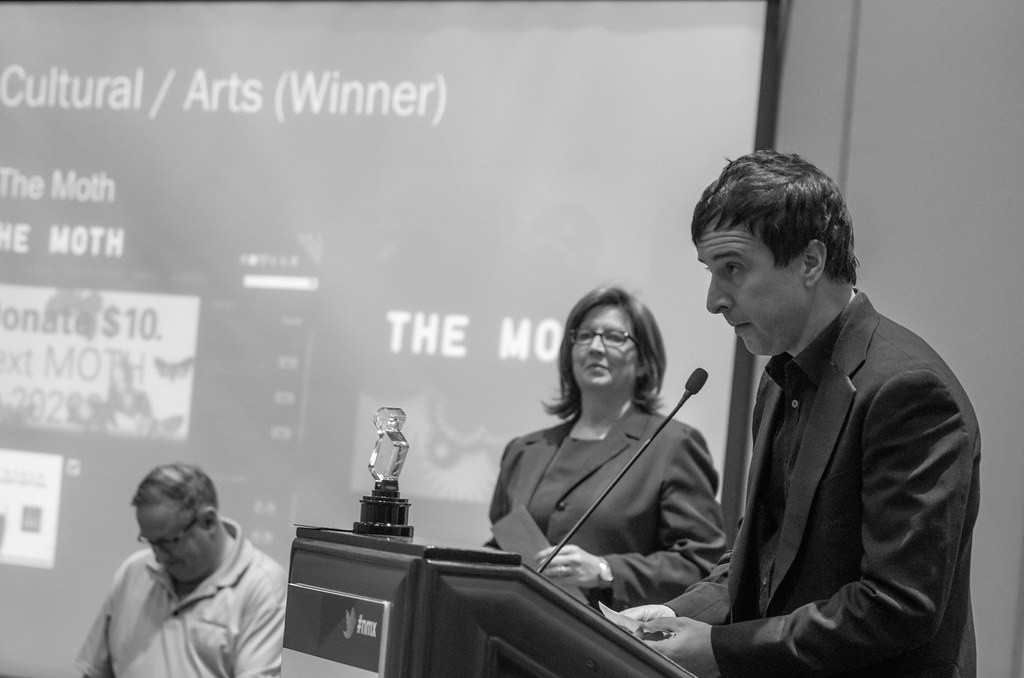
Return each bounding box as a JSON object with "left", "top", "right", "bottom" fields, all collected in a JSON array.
[{"left": 561, "top": 566, "right": 567, "bottom": 575}]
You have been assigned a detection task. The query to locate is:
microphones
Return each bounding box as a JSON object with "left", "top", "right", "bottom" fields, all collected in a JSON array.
[{"left": 539, "top": 366, "right": 708, "bottom": 573}]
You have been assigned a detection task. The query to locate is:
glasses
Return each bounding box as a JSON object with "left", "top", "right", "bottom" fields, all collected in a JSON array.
[
  {"left": 568, "top": 328, "right": 640, "bottom": 351},
  {"left": 137, "top": 517, "right": 197, "bottom": 548}
]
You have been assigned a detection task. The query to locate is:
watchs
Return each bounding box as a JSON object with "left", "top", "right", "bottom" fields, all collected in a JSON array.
[{"left": 598, "top": 557, "right": 613, "bottom": 590}]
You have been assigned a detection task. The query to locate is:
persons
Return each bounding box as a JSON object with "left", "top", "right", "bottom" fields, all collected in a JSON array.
[
  {"left": 476, "top": 285, "right": 726, "bottom": 613},
  {"left": 614, "top": 149, "right": 980, "bottom": 678},
  {"left": 74, "top": 465, "right": 287, "bottom": 678}
]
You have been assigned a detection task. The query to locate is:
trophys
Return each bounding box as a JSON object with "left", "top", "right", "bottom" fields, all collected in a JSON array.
[{"left": 354, "top": 408, "right": 417, "bottom": 539}]
